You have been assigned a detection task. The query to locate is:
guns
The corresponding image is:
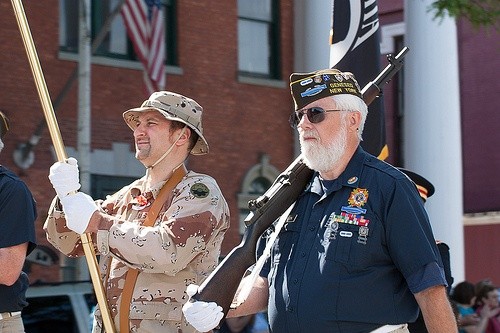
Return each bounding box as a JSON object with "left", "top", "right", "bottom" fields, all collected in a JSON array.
[{"left": 188, "top": 46, "right": 410, "bottom": 330}]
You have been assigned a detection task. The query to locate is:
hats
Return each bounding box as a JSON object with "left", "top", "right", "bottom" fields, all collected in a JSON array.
[
  {"left": 122, "top": 91, "right": 210, "bottom": 155},
  {"left": 289, "top": 69, "right": 367, "bottom": 112},
  {"left": 396, "top": 167, "right": 435, "bottom": 203},
  {"left": 0, "top": 112, "right": 9, "bottom": 138}
]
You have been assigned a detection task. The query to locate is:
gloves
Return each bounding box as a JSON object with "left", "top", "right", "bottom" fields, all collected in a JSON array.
[
  {"left": 60, "top": 192, "right": 100, "bottom": 235},
  {"left": 48, "top": 157, "right": 81, "bottom": 199},
  {"left": 181, "top": 284, "right": 225, "bottom": 333}
]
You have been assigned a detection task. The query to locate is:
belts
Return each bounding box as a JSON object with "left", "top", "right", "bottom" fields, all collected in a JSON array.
[
  {"left": 369, "top": 323, "right": 408, "bottom": 333},
  {"left": 0, "top": 310, "right": 21, "bottom": 321}
]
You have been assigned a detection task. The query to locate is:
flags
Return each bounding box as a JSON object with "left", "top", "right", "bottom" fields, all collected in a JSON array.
[
  {"left": 330, "top": 1, "right": 389, "bottom": 161},
  {"left": 121, "top": 1, "right": 166, "bottom": 94}
]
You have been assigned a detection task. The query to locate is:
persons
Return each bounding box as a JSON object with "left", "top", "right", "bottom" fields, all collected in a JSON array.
[
  {"left": 449, "top": 279, "right": 500, "bottom": 333},
  {"left": 398, "top": 167, "right": 454, "bottom": 333},
  {"left": 42, "top": 91, "right": 230, "bottom": 333},
  {"left": 0, "top": 110, "right": 36, "bottom": 333},
  {"left": 182, "top": 70, "right": 459, "bottom": 333}
]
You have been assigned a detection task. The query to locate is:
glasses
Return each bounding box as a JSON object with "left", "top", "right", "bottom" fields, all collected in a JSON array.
[{"left": 287, "top": 106, "right": 351, "bottom": 130}]
